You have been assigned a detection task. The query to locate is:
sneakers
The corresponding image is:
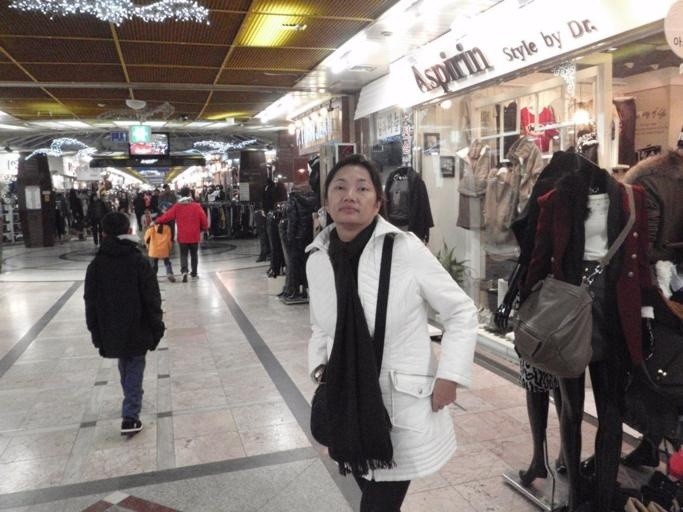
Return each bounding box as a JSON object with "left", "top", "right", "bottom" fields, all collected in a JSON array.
[
  {"left": 122, "top": 420, "right": 142, "bottom": 434},
  {"left": 168, "top": 271, "right": 197, "bottom": 282}
]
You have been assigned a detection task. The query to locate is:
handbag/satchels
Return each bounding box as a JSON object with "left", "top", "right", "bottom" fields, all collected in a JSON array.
[
  {"left": 513, "top": 277, "right": 593, "bottom": 379},
  {"left": 621, "top": 321, "right": 683, "bottom": 438}
]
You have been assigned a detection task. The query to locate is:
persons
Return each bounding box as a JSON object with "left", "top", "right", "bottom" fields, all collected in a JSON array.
[
  {"left": 148, "top": 186, "right": 208, "bottom": 281},
  {"left": 54, "top": 188, "right": 106, "bottom": 246},
  {"left": 452, "top": 135, "right": 547, "bottom": 245},
  {"left": 306, "top": 152, "right": 476, "bottom": 511},
  {"left": 383, "top": 165, "right": 434, "bottom": 246},
  {"left": 144, "top": 212, "right": 175, "bottom": 282},
  {"left": 491, "top": 131, "right": 683, "bottom": 512},
  {"left": 254, "top": 186, "right": 320, "bottom": 301},
  {"left": 157, "top": 184, "right": 176, "bottom": 240},
  {"left": 83, "top": 209, "right": 164, "bottom": 436},
  {"left": 132, "top": 188, "right": 159, "bottom": 231}
]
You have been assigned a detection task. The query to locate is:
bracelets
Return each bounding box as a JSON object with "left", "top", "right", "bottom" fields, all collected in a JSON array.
[{"left": 313, "top": 367, "right": 325, "bottom": 379}]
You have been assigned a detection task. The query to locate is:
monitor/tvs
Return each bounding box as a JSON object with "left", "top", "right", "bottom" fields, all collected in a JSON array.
[
  {"left": 128, "top": 124, "right": 152, "bottom": 145},
  {"left": 128, "top": 133, "right": 170, "bottom": 159}
]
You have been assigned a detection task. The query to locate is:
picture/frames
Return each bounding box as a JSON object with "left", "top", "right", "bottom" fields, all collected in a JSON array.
[{"left": 424, "top": 132, "right": 456, "bottom": 177}]
